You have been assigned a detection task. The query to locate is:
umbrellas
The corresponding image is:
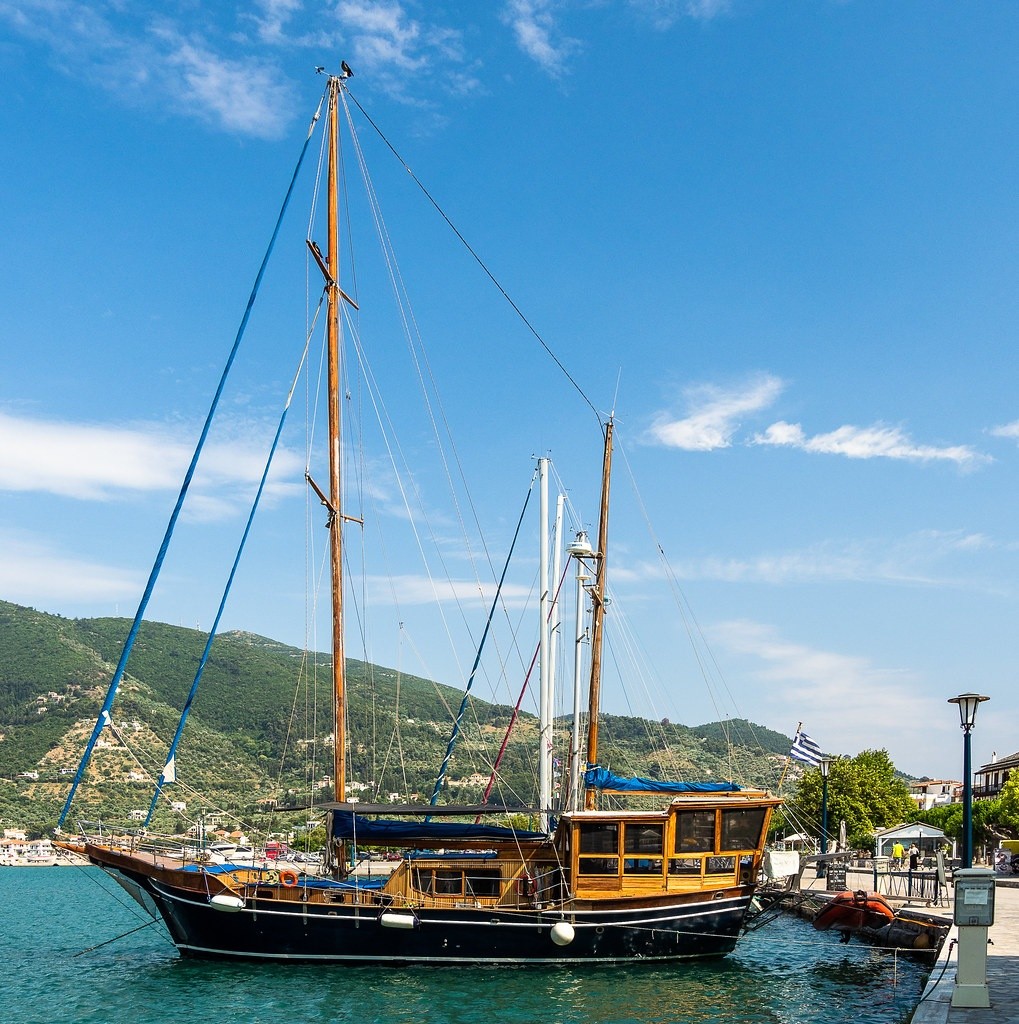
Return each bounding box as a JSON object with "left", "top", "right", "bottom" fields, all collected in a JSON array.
[{"left": 782, "top": 832, "right": 809, "bottom": 843}]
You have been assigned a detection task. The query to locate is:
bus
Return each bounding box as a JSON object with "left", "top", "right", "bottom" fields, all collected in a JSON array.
[{"left": 205, "top": 845, "right": 256, "bottom": 861}]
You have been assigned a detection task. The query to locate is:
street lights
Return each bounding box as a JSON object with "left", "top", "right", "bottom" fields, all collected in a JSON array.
[
  {"left": 919, "top": 827, "right": 923, "bottom": 859},
  {"left": 814, "top": 756, "right": 837, "bottom": 879},
  {"left": 947, "top": 691, "right": 991, "bottom": 868}
]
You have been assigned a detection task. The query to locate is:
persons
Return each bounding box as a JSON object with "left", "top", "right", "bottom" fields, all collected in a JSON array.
[
  {"left": 903, "top": 843, "right": 919, "bottom": 872},
  {"left": 856, "top": 850, "right": 873, "bottom": 868},
  {"left": 892, "top": 840, "right": 906, "bottom": 871}
]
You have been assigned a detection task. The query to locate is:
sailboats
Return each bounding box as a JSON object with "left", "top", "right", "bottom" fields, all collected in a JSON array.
[{"left": 46, "top": 59, "right": 791, "bottom": 968}]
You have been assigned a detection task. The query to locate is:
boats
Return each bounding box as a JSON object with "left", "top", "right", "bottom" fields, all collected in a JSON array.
[{"left": 811, "top": 889, "right": 895, "bottom": 934}]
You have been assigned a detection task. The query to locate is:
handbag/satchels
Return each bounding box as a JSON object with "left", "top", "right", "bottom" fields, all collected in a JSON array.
[{"left": 916, "top": 851, "right": 919, "bottom": 857}]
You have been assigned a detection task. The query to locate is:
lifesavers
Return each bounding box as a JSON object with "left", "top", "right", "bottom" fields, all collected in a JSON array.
[
  {"left": 514, "top": 874, "right": 538, "bottom": 896},
  {"left": 279, "top": 871, "right": 298, "bottom": 888}
]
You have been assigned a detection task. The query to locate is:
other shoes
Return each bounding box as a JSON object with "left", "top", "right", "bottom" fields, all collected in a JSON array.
[
  {"left": 899, "top": 867, "right": 901, "bottom": 871},
  {"left": 893, "top": 869, "right": 897, "bottom": 871}
]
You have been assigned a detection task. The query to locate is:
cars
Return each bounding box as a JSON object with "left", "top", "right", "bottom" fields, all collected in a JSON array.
[
  {"left": 388, "top": 852, "right": 402, "bottom": 862},
  {"left": 369, "top": 852, "right": 384, "bottom": 862},
  {"left": 277, "top": 850, "right": 322, "bottom": 863},
  {"left": 354, "top": 852, "right": 369, "bottom": 860}
]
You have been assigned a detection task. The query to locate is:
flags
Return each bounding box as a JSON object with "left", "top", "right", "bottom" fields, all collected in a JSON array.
[{"left": 788, "top": 732, "right": 822, "bottom": 767}]
